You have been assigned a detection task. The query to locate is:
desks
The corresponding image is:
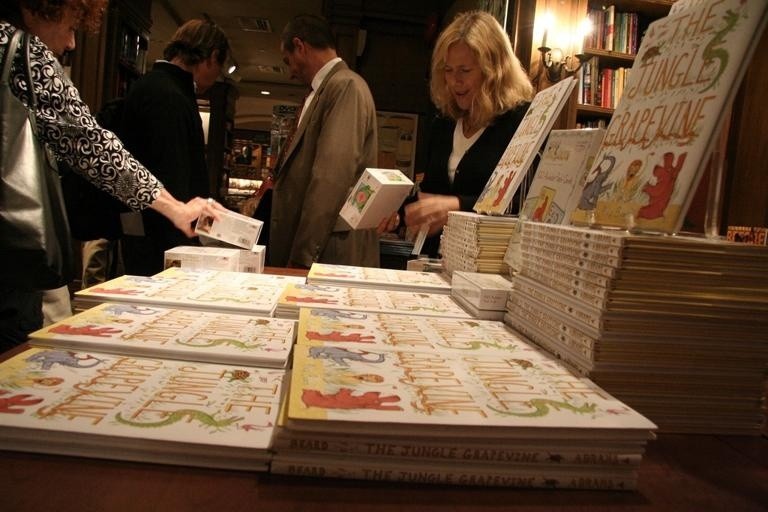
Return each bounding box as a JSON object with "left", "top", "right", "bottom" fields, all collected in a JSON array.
[{"left": 1, "top": 263, "right": 768, "bottom": 512}]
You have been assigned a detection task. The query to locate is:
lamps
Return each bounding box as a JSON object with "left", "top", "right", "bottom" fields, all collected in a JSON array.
[{"left": 537, "top": 8, "right": 590, "bottom": 84}]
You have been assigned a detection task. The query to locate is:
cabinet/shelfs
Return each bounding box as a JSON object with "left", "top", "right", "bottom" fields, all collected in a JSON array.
[{"left": 566, "top": 1, "right": 676, "bottom": 128}]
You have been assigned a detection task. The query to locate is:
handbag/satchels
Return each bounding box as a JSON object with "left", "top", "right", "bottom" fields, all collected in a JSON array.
[{"left": 0, "top": 29, "right": 71, "bottom": 276}]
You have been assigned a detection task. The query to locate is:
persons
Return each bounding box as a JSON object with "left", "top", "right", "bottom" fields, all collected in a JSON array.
[
  {"left": 85, "top": 18, "right": 232, "bottom": 277},
  {"left": 1, "top": 1, "right": 227, "bottom": 353},
  {"left": 376, "top": 10, "right": 548, "bottom": 264},
  {"left": 267, "top": 12, "right": 380, "bottom": 270}
]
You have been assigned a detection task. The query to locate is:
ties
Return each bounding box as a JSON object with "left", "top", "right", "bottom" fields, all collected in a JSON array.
[{"left": 274, "top": 87, "right": 313, "bottom": 174}]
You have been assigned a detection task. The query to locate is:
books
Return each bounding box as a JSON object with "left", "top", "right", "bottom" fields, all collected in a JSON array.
[
  {"left": 0, "top": 262, "right": 658, "bottom": 489},
  {"left": 439, "top": 1, "right": 767, "bottom": 437}
]
